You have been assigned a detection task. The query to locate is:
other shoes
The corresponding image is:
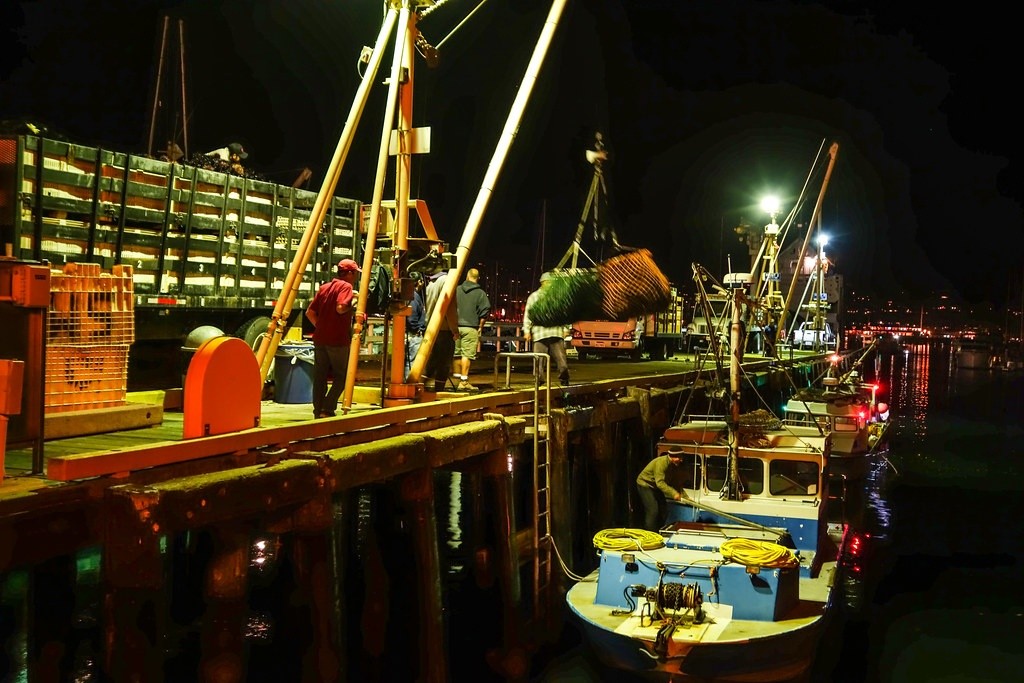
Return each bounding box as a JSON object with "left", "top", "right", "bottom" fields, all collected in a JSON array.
[
  {"left": 560, "top": 381, "right": 569, "bottom": 386},
  {"left": 314, "top": 409, "right": 335, "bottom": 419},
  {"left": 457, "top": 379, "right": 479, "bottom": 392}
]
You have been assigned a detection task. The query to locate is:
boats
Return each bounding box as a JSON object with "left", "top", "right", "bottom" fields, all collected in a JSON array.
[
  {"left": 782, "top": 344, "right": 893, "bottom": 485},
  {"left": 562, "top": 251, "right": 852, "bottom": 683}
]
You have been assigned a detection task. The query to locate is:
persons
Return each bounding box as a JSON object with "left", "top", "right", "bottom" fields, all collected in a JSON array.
[
  {"left": 204, "top": 142, "right": 247, "bottom": 166},
  {"left": 765, "top": 318, "right": 777, "bottom": 358},
  {"left": 636, "top": 446, "right": 684, "bottom": 531},
  {"left": 406, "top": 268, "right": 490, "bottom": 391},
  {"left": 306, "top": 259, "right": 362, "bottom": 419},
  {"left": 523, "top": 273, "right": 569, "bottom": 386}
]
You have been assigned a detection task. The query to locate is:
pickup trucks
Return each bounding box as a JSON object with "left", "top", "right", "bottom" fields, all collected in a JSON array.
[{"left": 792, "top": 322, "right": 836, "bottom": 350}]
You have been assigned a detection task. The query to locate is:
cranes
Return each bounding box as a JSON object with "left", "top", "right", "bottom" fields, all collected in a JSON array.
[{"left": 741, "top": 141, "right": 838, "bottom": 352}]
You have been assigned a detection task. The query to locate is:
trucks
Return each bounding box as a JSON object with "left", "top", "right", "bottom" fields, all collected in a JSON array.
[
  {"left": 685, "top": 292, "right": 764, "bottom": 354},
  {"left": 571, "top": 286, "right": 685, "bottom": 363},
  {"left": 0, "top": 134, "right": 393, "bottom": 384}
]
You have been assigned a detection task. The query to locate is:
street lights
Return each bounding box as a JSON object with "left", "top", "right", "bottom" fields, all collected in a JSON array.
[
  {"left": 764, "top": 197, "right": 781, "bottom": 295},
  {"left": 817, "top": 235, "right": 828, "bottom": 293}
]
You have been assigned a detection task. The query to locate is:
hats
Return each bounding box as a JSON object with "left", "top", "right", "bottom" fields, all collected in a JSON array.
[
  {"left": 338, "top": 258, "right": 362, "bottom": 274},
  {"left": 668, "top": 445, "right": 684, "bottom": 458},
  {"left": 540, "top": 272, "right": 552, "bottom": 282}
]
